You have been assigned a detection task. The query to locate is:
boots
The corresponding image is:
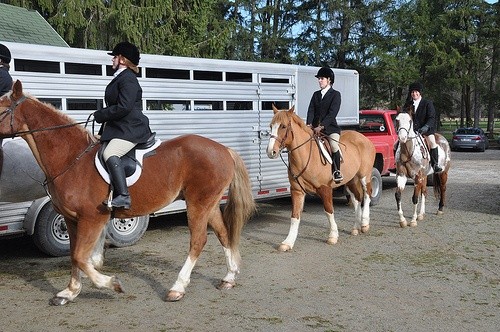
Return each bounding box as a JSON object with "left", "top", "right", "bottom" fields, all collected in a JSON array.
[
  {"left": 331, "top": 150, "right": 342, "bottom": 184},
  {"left": 102, "top": 155, "right": 132, "bottom": 210},
  {"left": 429, "top": 146, "right": 443, "bottom": 173}
]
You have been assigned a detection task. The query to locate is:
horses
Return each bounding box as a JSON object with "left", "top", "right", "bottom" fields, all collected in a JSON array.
[
  {"left": 0, "top": 79, "right": 255, "bottom": 307},
  {"left": 265, "top": 103, "right": 377, "bottom": 253},
  {"left": 392, "top": 105, "right": 451, "bottom": 228}
]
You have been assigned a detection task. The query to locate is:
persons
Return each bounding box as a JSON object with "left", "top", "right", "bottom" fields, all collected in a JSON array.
[
  {"left": 0, "top": 42, "right": 15, "bottom": 98},
  {"left": 93, "top": 42, "right": 151, "bottom": 209},
  {"left": 403, "top": 83, "right": 443, "bottom": 172},
  {"left": 306, "top": 66, "right": 342, "bottom": 183}
]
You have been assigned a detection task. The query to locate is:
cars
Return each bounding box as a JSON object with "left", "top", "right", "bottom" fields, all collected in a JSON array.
[{"left": 449, "top": 127, "right": 488, "bottom": 153}]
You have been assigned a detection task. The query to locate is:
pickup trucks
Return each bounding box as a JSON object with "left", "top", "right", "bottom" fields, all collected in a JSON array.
[{"left": 354, "top": 110, "right": 437, "bottom": 206}]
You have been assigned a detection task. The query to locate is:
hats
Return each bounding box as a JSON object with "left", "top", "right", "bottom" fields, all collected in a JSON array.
[
  {"left": 0, "top": 43, "right": 11, "bottom": 63},
  {"left": 315, "top": 67, "right": 335, "bottom": 78},
  {"left": 107, "top": 41, "right": 141, "bottom": 66},
  {"left": 409, "top": 82, "right": 423, "bottom": 92}
]
